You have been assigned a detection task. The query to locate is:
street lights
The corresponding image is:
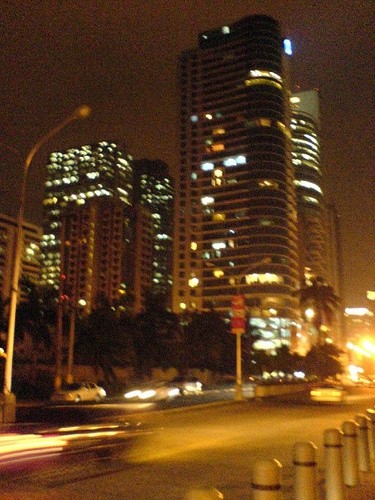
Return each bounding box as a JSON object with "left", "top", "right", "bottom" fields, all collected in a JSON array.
[
  {"left": 0, "top": 106, "right": 92, "bottom": 422},
  {"left": 233, "top": 257, "right": 270, "bottom": 404}
]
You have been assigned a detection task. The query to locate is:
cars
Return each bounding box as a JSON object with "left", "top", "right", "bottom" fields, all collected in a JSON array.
[
  {"left": 123, "top": 376, "right": 202, "bottom": 401},
  {"left": 49, "top": 381, "right": 106, "bottom": 405},
  {"left": 310, "top": 382, "right": 343, "bottom": 402}
]
